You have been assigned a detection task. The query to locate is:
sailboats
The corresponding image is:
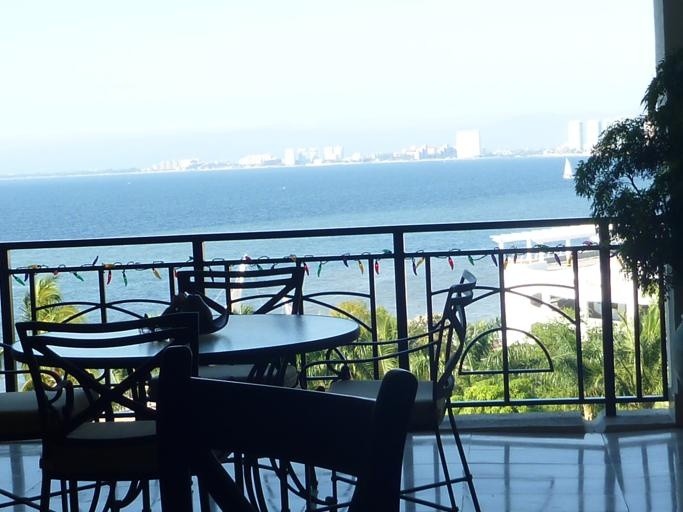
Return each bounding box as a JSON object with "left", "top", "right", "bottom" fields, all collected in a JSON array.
[
  {"left": 209, "top": 252, "right": 293, "bottom": 316},
  {"left": 560, "top": 156, "right": 574, "bottom": 180}
]
